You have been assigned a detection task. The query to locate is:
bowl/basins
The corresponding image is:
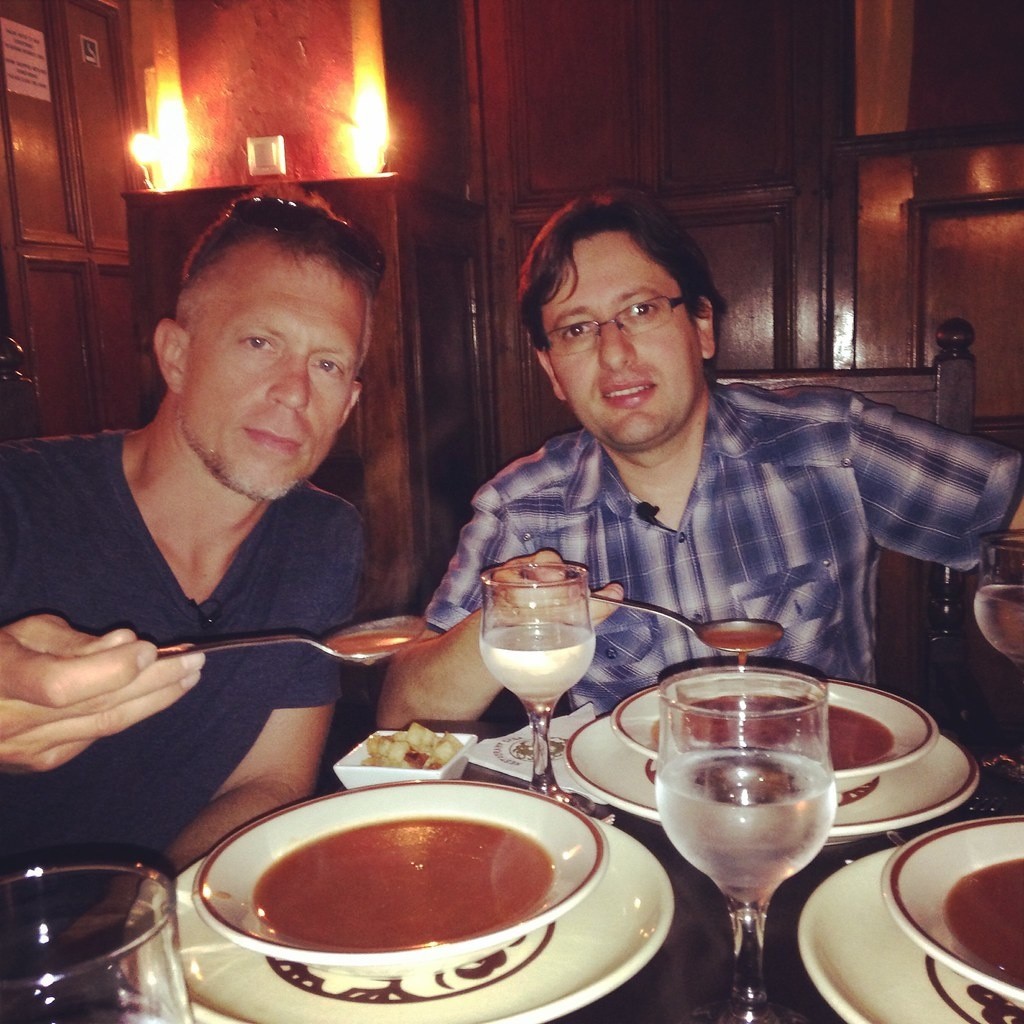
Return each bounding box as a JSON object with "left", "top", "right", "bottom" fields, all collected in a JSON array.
[
  {"left": 611, "top": 679, "right": 941, "bottom": 793},
  {"left": 333, "top": 730, "right": 478, "bottom": 789},
  {"left": 880, "top": 815, "right": 1024, "bottom": 1011},
  {"left": 192, "top": 780, "right": 610, "bottom": 978}
]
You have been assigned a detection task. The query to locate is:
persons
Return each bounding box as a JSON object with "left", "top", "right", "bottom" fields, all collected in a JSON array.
[
  {"left": 375, "top": 197, "right": 1024, "bottom": 731},
  {"left": 0, "top": 181, "right": 385, "bottom": 875}
]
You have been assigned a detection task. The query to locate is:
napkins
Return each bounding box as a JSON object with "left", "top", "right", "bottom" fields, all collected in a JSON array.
[{"left": 468, "top": 702, "right": 606, "bottom": 806}]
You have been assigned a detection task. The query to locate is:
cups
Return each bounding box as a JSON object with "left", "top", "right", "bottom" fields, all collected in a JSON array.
[
  {"left": 973, "top": 530, "right": 1024, "bottom": 671},
  {"left": 0, "top": 861, "right": 194, "bottom": 1024}
]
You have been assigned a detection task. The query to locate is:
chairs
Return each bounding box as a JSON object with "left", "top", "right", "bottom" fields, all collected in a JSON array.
[{"left": 708, "top": 321, "right": 976, "bottom": 709}]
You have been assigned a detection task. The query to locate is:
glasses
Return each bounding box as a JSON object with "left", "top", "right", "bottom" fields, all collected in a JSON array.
[
  {"left": 538, "top": 296, "right": 684, "bottom": 354},
  {"left": 181, "top": 197, "right": 386, "bottom": 298}
]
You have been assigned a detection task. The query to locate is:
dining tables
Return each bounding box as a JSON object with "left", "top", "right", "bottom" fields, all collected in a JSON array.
[{"left": 343, "top": 720, "right": 1023, "bottom": 1024}]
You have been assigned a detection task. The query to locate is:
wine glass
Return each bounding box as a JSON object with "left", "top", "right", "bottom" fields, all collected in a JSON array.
[
  {"left": 479, "top": 561, "right": 596, "bottom": 816},
  {"left": 654, "top": 665, "right": 838, "bottom": 1024}
]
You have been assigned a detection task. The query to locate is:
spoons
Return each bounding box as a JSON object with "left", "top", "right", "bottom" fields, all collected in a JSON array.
[
  {"left": 518, "top": 569, "right": 784, "bottom": 653},
  {"left": 156, "top": 614, "right": 426, "bottom": 660}
]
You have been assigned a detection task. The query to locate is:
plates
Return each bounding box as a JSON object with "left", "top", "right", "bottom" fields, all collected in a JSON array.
[
  {"left": 565, "top": 713, "right": 979, "bottom": 846},
  {"left": 797, "top": 846, "right": 1024, "bottom": 1024},
  {"left": 175, "top": 816, "right": 675, "bottom": 1024}
]
